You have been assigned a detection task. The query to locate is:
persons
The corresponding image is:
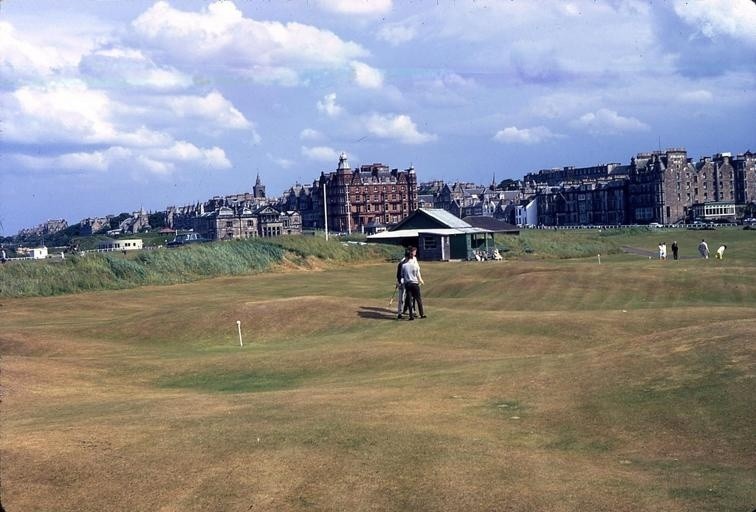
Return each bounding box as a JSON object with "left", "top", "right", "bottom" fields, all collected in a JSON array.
[
  {"left": 715, "top": 245, "right": 728, "bottom": 260},
  {"left": 397, "top": 245, "right": 418, "bottom": 320},
  {"left": 662, "top": 242, "right": 668, "bottom": 259},
  {"left": 658, "top": 242, "right": 666, "bottom": 260},
  {"left": 698, "top": 238, "right": 711, "bottom": 260},
  {"left": 399, "top": 248, "right": 427, "bottom": 320},
  {"left": 671, "top": 240, "right": 680, "bottom": 261}
]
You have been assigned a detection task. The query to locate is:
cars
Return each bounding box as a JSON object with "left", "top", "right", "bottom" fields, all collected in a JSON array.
[
  {"left": 648, "top": 222, "right": 664, "bottom": 229},
  {"left": 164, "top": 232, "right": 213, "bottom": 247}
]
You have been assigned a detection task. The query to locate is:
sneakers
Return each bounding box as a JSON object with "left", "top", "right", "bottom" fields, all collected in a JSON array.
[{"left": 398, "top": 312, "right": 427, "bottom": 320}]
